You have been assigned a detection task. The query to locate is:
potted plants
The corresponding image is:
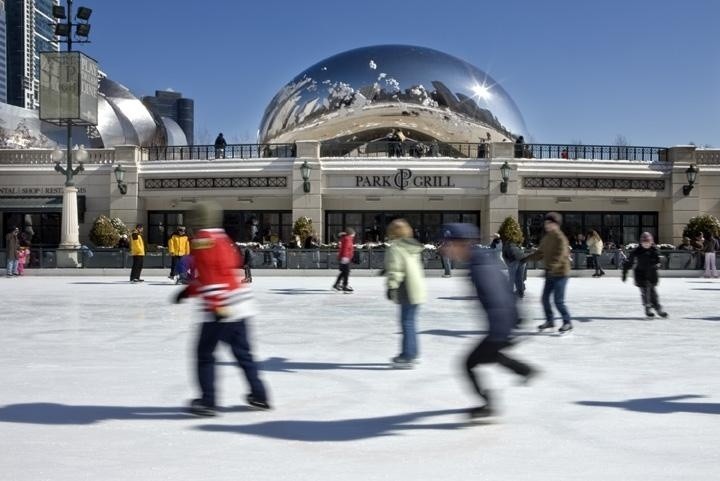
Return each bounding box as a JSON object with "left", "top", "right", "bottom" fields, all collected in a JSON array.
[
  {"left": 89, "top": 216, "right": 124, "bottom": 267},
  {"left": 287, "top": 216, "right": 320, "bottom": 268},
  {"left": 682, "top": 217, "right": 719, "bottom": 268},
  {"left": 489, "top": 216, "right": 533, "bottom": 268},
  {"left": 112, "top": 216, "right": 130, "bottom": 260},
  {"left": 248, "top": 216, "right": 286, "bottom": 267}
]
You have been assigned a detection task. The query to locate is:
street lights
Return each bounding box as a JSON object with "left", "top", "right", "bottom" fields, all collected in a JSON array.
[{"left": 39, "top": 0, "right": 98, "bottom": 185}]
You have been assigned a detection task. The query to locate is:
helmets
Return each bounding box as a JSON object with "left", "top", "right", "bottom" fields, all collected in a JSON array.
[{"left": 191, "top": 200, "right": 223, "bottom": 228}]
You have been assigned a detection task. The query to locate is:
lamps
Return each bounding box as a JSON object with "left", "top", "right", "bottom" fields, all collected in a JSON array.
[
  {"left": 300, "top": 160, "right": 312, "bottom": 192},
  {"left": 115, "top": 164, "right": 127, "bottom": 194},
  {"left": 500, "top": 161, "right": 510, "bottom": 192},
  {"left": 683, "top": 165, "right": 697, "bottom": 195}
]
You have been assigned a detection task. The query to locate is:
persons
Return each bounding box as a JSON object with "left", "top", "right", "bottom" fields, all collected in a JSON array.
[
  {"left": 291, "top": 143, "right": 296, "bottom": 157},
  {"left": 167, "top": 227, "right": 356, "bottom": 292},
  {"left": 439, "top": 238, "right": 452, "bottom": 275},
  {"left": 170, "top": 201, "right": 268, "bottom": 409},
  {"left": 561, "top": 150, "right": 568, "bottom": 158},
  {"left": 479, "top": 139, "right": 486, "bottom": 158},
  {"left": 514, "top": 136, "right": 535, "bottom": 158},
  {"left": 383, "top": 217, "right": 425, "bottom": 363},
  {"left": 263, "top": 145, "right": 273, "bottom": 157},
  {"left": 386, "top": 128, "right": 440, "bottom": 157},
  {"left": 490, "top": 211, "right": 720, "bottom": 332},
  {"left": 118, "top": 234, "right": 130, "bottom": 249},
  {"left": 442, "top": 223, "right": 540, "bottom": 418},
  {"left": 130, "top": 224, "right": 146, "bottom": 282},
  {"left": 5, "top": 227, "right": 30, "bottom": 276},
  {"left": 214, "top": 133, "right": 227, "bottom": 159}
]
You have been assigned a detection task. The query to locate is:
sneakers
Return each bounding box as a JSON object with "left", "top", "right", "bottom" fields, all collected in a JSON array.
[
  {"left": 646, "top": 307, "right": 654, "bottom": 316},
  {"left": 540, "top": 320, "right": 553, "bottom": 328},
  {"left": 559, "top": 323, "right": 572, "bottom": 330}
]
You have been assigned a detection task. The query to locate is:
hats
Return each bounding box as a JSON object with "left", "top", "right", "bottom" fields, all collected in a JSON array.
[
  {"left": 435, "top": 221, "right": 480, "bottom": 241},
  {"left": 544, "top": 212, "right": 563, "bottom": 226},
  {"left": 639, "top": 231, "right": 653, "bottom": 243},
  {"left": 386, "top": 218, "right": 412, "bottom": 236}
]
